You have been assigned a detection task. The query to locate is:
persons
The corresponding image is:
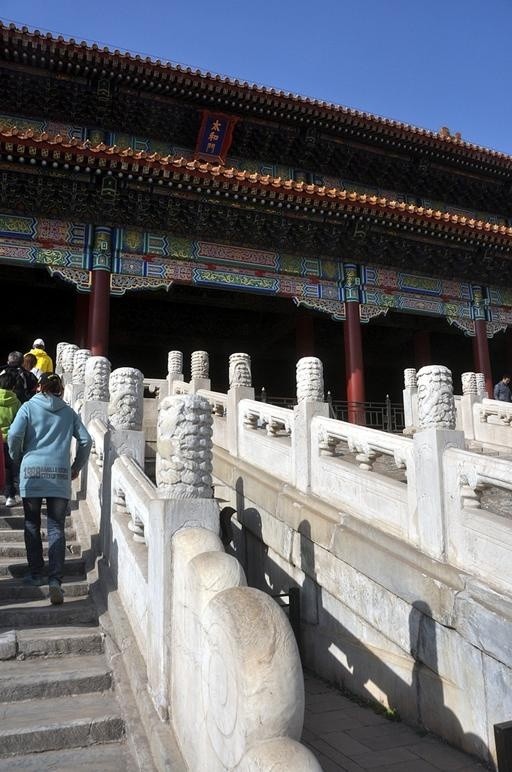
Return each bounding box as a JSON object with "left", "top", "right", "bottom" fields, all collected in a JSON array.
[
  {"left": 6, "top": 372, "right": 94, "bottom": 605},
  {"left": 21, "top": 354, "right": 42, "bottom": 380},
  {"left": 26, "top": 339, "right": 56, "bottom": 372},
  {"left": 0, "top": 373, "right": 25, "bottom": 509},
  {"left": 1, "top": 351, "right": 39, "bottom": 403},
  {"left": 492, "top": 373, "right": 511, "bottom": 404}
]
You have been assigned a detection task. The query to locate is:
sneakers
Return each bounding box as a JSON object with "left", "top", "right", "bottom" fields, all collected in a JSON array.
[
  {"left": 5, "top": 496, "right": 18, "bottom": 507},
  {"left": 48, "top": 578, "right": 64, "bottom": 604},
  {"left": 23, "top": 574, "right": 43, "bottom": 585}
]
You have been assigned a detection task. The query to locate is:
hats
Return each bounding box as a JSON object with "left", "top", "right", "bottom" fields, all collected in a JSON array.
[{"left": 33, "top": 339, "right": 45, "bottom": 346}]
